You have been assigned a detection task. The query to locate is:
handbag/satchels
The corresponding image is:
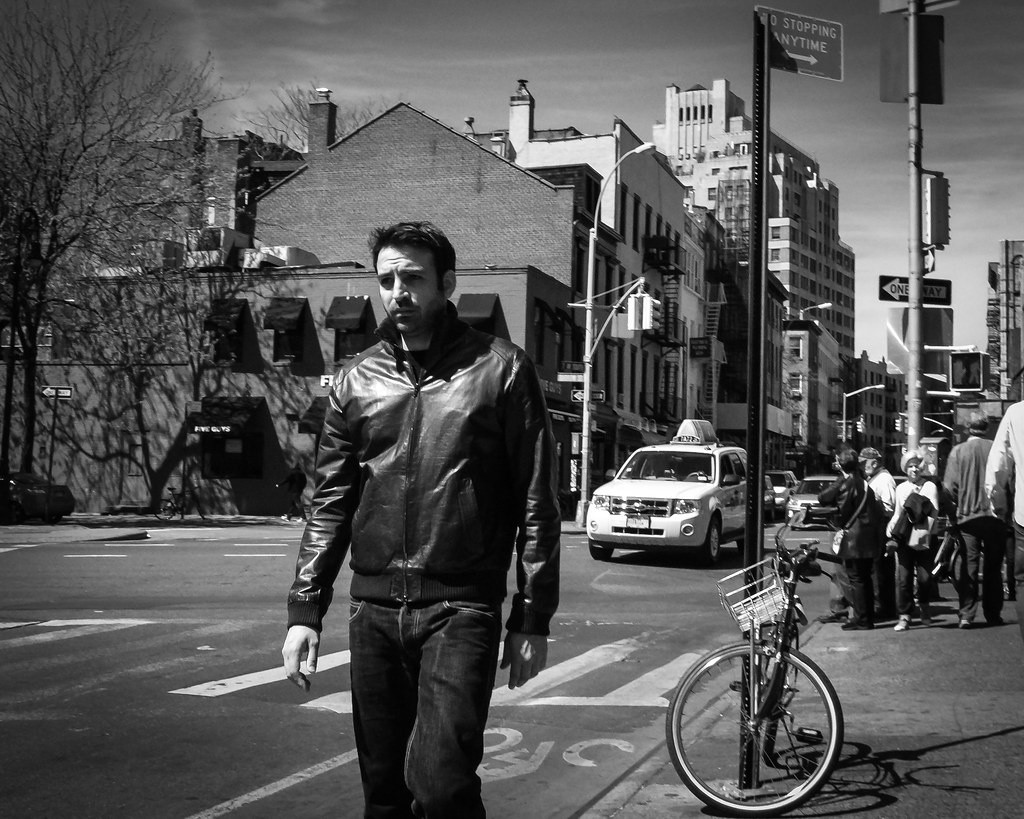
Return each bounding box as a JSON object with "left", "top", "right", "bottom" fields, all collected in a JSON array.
[
  {"left": 831, "top": 528, "right": 855, "bottom": 558},
  {"left": 907, "top": 516, "right": 935, "bottom": 551}
]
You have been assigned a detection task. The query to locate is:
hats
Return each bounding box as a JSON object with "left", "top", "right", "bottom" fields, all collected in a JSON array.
[
  {"left": 901, "top": 450, "right": 925, "bottom": 474},
  {"left": 859, "top": 447, "right": 883, "bottom": 460}
]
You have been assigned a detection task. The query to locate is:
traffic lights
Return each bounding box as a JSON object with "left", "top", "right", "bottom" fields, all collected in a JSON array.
[
  {"left": 904, "top": 420, "right": 908, "bottom": 434},
  {"left": 947, "top": 353, "right": 991, "bottom": 393},
  {"left": 856, "top": 419, "right": 865, "bottom": 435},
  {"left": 570, "top": 389, "right": 605, "bottom": 404},
  {"left": 642, "top": 296, "right": 662, "bottom": 332},
  {"left": 896, "top": 418, "right": 903, "bottom": 432}
]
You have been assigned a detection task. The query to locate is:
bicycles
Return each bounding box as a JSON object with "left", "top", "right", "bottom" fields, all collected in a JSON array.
[
  {"left": 663, "top": 509, "right": 845, "bottom": 817},
  {"left": 913, "top": 516, "right": 985, "bottom": 599},
  {"left": 155, "top": 484, "right": 206, "bottom": 521}
]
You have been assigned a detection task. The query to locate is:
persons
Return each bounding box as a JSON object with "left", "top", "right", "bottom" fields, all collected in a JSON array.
[
  {"left": 983, "top": 400, "right": 1024, "bottom": 638},
  {"left": 282, "top": 222, "right": 560, "bottom": 819},
  {"left": 661, "top": 461, "right": 679, "bottom": 478},
  {"left": 818, "top": 410, "right": 1015, "bottom": 631}
]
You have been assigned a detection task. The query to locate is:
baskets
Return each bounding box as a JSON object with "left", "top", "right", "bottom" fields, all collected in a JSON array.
[{"left": 717, "top": 555, "right": 788, "bottom": 634}]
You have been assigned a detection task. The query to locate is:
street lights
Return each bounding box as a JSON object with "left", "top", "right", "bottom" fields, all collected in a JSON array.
[
  {"left": 578, "top": 140, "right": 656, "bottom": 530},
  {"left": 842, "top": 385, "right": 888, "bottom": 442},
  {"left": 798, "top": 303, "right": 832, "bottom": 436}
]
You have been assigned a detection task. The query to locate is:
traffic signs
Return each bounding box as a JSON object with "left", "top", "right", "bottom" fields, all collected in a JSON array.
[
  {"left": 879, "top": 275, "right": 953, "bottom": 307},
  {"left": 41, "top": 385, "right": 72, "bottom": 399},
  {"left": 924, "top": 247, "right": 937, "bottom": 274},
  {"left": 764, "top": 7, "right": 845, "bottom": 83}
]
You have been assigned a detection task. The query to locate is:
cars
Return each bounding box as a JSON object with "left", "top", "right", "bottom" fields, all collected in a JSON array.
[
  {"left": 786, "top": 475, "right": 840, "bottom": 527},
  {"left": 1, "top": 470, "right": 76, "bottom": 524}
]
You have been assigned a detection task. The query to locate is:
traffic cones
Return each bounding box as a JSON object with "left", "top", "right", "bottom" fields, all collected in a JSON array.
[{"left": 764, "top": 471, "right": 799, "bottom": 519}]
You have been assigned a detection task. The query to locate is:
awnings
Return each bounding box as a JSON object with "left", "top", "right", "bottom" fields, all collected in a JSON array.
[
  {"left": 457, "top": 294, "right": 499, "bottom": 333},
  {"left": 325, "top": 296, "right": 370, "bottom": 330},
  {"left": 204, "top": 298, "right": 247, "bottom": 334},
  {"left": 299, "top": 396, "right": 328, "bottom": 434},
  {"left": 188, "top": 396, "right": 266, "bottom": 437},
  {"left": 264, "top": 297, "right": 308, "bottom": 330}
]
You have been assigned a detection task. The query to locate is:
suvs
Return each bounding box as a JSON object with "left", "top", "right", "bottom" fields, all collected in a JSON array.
[{"left": 584, "top": 419, "right": 747, "bottom": 564}]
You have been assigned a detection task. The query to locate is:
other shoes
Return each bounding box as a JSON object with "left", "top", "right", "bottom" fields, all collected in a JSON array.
[
  {"left": 841, "top": 621, "right": 875, "bottom": 631},
  {"left": 959, "top": 619, "right": 971, "bottom": 629},
  {"left": 894, "top": 614, "right": 911, "bottom": 631}
]
